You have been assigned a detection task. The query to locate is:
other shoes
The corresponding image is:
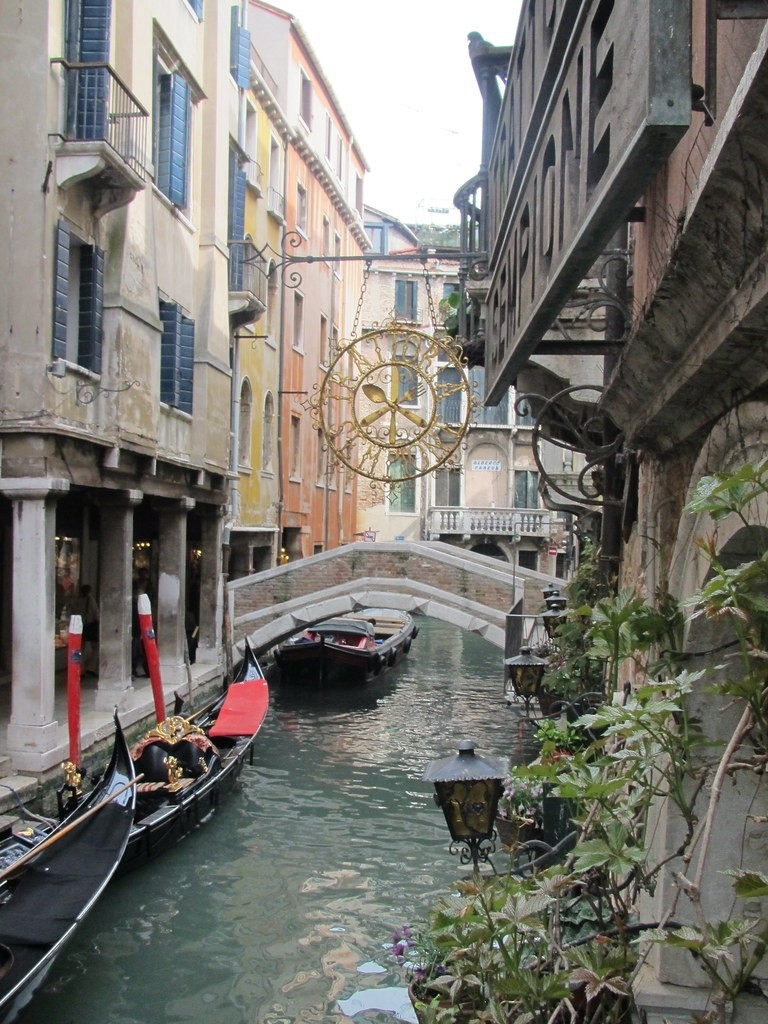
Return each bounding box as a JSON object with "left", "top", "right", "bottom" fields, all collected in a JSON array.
[
  {"left": 81, "top": 668, "right": 98, "bottom": 680},
  {"left": 132, "top": 669, "right": 150, "bottom": 678}
]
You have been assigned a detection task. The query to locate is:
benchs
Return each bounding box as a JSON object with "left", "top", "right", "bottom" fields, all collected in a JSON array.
[{"left": 96, "top": 714, "right": 221, "bottom": 801}]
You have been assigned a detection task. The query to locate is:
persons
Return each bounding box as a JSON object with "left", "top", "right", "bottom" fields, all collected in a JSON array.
[
  {"left": 76, "top": 585, "right": 99, "bottom": 679},
  {"left": 125, "top": 552, "right": 199, "bottom": 679}
]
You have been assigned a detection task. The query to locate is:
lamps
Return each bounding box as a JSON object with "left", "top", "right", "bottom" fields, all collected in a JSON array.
[
  {"left": 503, "top": 645, "right": 547, "bottom": 718},
  {"left": 542, "top": 583, "right": 558, "bottom": 598},
  {"left": 544, "top": 591, "right": 566, "bottom": 610},
  {"left": 424, "top": 741, "right": 509, "bottom": 902},
  {"left": 540, "top": 603, "right": 565, "bottom": 639}
]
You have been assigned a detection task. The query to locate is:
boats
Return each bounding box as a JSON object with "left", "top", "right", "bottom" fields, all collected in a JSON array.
[
  {"left": 0, "top": 635, "right": 271, "bottom": 881},
  {"left": 273, "top": 607, "right": 419, "bottom": 689},
  {"left": 0, "top": 707, "right": 143, "bottom": 1010}
]
